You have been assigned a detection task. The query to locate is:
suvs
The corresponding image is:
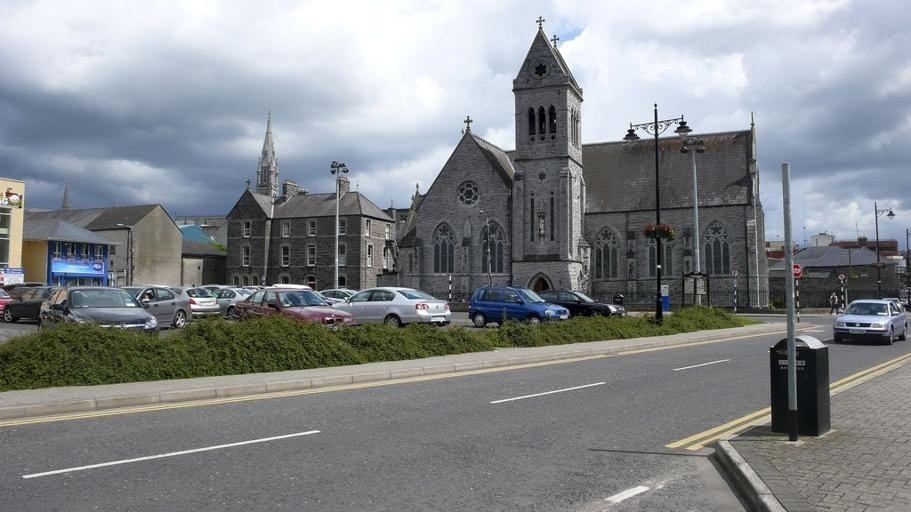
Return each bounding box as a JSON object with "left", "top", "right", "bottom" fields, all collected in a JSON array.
[{"left": 468, "top": 284, "right": 570, "bottom": 328}]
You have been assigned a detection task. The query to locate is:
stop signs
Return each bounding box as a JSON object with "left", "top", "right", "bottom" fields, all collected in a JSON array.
[{"left": 791, "top": 262, "right": 802, "bottom": 280}]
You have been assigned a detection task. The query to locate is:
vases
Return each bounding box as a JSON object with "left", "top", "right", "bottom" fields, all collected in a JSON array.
[{"left": 650, "top": 232, "right": 668, "bottom": 239}]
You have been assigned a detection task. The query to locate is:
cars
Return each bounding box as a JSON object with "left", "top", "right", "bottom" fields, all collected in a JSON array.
[
  {"left": 39, "top": 285, "right": 159, "bottom": 341},
  {"left": 0, "top": 286, "right": 54, "bottom": 324},
  {"left": 168, "top": 284, "right": 263, "bottom": 318},
  {"left": 833, "top": 298, "right": 909, "bottom": 345},
  {"left": 537, "top": 290, "right": 626, "bottom": 320},
  {"left": 234, "top": 286, "right": 353, "bottom": 330},
  {"left": 318, "top": 286, "right": 452, "bottom": 329},
  {"left": 121, "top": 287, "right": 191, "bottom": 329}
]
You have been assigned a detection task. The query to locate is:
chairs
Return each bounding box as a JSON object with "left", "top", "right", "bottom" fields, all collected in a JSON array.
[
  {"left": 94, "top": 295, "right": 113, "bottom": 305},
  {"left": 32, "top": 291, "right": 42, "bottom": 300},
  {"left": 73, "top": 292, "right": 85, "bottom": 305},
  {"left": 140, "top": 294, "right": 169, "bottom": 301},
  {"left": 283, "top": 293, "right": 301, "bottom": 305}
]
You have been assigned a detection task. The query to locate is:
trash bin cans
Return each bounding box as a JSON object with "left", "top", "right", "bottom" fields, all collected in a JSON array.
[
  {"left": 613, "top": 293, "right": 624, "bottom": 306},
  {"left": 769, "top": 336, "right": 830, "bottom": 437}
]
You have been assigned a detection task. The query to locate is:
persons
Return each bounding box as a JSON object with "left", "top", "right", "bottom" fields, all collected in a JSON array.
[{"left": 829, "top": 291, "right": 840, "bottom": 315}]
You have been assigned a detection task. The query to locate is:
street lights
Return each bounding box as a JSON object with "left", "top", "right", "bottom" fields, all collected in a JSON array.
[
  {"left": 330, "top": 161, "right": 349, "bottom": 288},
  {"left": 679, "top": 137, "right": 705, "bottom": 307},
  {"left": 622, "top": 102, "right": 692, "bottom": 327},
  {"left": 873, "top": 202, "right": 896, "bottom": 298}
]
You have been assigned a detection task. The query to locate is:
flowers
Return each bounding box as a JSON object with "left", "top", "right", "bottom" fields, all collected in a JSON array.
[{"left": 644, "top": 222, "right": 675, "bottom": 241}]
